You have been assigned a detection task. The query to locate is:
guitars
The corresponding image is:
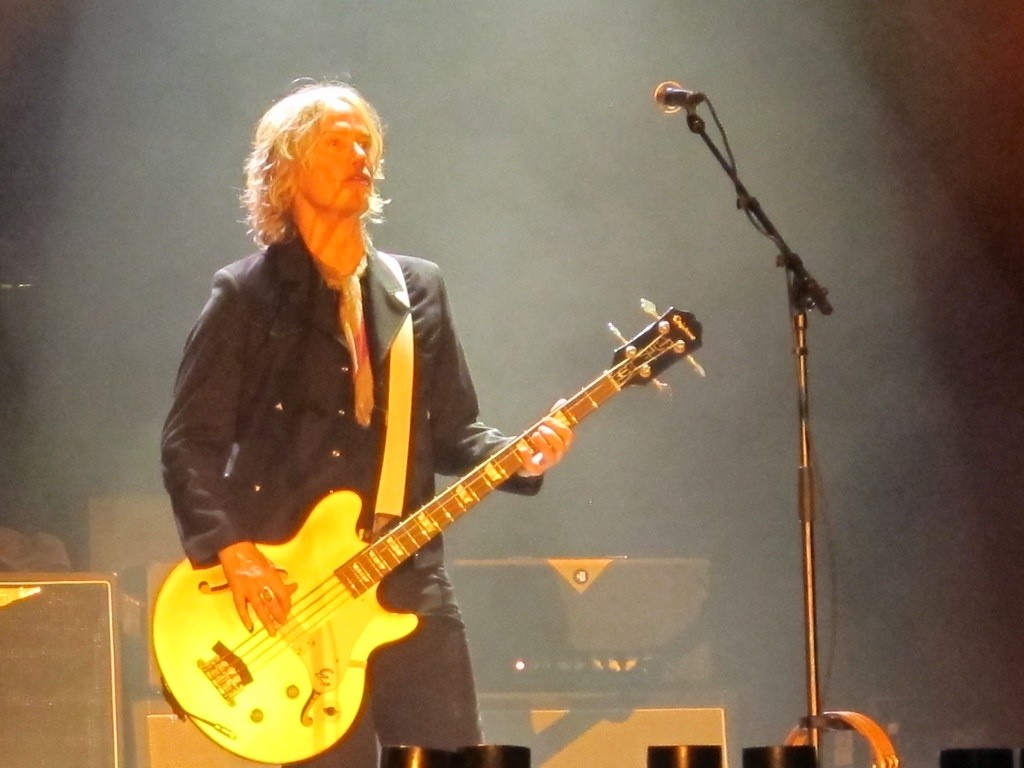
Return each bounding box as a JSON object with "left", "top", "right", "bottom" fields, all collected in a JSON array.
[{"left": 150, "top": 298, "right": 709, "bottom": 766}]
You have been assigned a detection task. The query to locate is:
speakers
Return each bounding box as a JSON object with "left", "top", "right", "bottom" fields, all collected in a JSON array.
[
  {"left": 0, "top": 572, "right": 126, "bottom": 768},
  {"left": 126, "top": 698, "right": 283, "bottom": 768}
]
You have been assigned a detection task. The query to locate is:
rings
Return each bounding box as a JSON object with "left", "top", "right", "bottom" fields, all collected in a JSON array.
[{"left": 259, "top": 586, "right": 275, "bottom": 604}]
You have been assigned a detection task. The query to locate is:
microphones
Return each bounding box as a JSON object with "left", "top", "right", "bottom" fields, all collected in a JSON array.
[{"left": 654, "top": 81, "right": 705, "bottom": 113}]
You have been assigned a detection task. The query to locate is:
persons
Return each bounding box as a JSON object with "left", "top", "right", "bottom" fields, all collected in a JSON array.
[{"left": 158, "top": 84, "right": 573, "bottom": 767}]
